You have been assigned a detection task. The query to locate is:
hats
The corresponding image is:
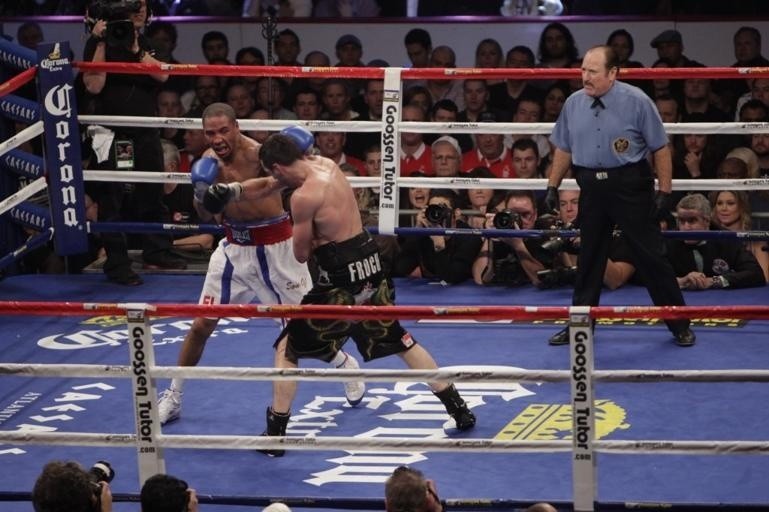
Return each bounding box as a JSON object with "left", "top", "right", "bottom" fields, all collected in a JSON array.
[
  {"left": 433, "top": 137, "right": 462, "bottom": 160},
  {"left": 475, "top": 111, "right": 500, "bottom": 124},
  {"left": 649, "top": 30, "right": 681, "bottom": 48}
]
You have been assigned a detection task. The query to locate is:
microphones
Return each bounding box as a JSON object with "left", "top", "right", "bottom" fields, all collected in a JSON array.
[{"left": 145, "top": 7, "right": 154, "bottom": 28}]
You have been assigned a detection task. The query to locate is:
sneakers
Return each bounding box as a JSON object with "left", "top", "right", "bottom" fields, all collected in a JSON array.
[
  {"left": 335, "top": 351, "right": 365, "bottom": 406},
  {"left": 158, "top": 387, "right": 185, "bottom": 426},
  {"left": 454, "top": 408, "right": 477, "bottom": 431},
  {"left": 107, "top": 267, "right": 144, "bottom": 285},
  {"left": 255, "top": 432, "right": 285, "bottom": 458},
  {"left": 142, "top": 247, "right": 188, "bottom": 270}
]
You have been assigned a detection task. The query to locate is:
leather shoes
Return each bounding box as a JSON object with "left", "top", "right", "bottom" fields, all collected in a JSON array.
[
  {"left": 675, "top": 327, "right": 696, "bottom": 345},
  {"left": 549, "top": 325, "right": 568, "bottom": 344}
]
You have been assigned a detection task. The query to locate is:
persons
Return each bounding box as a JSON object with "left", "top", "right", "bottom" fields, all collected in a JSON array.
[
  {"left": 155, "top": 103, "right": 365, "bottom": 425},
  {"left": 504, "top": 23, "right": 768, "bottom": 228},
  {"left": 628, "top": 192, "right": 766, "bottom": 290},
  {"left": 140, "top": 474, "right": 198, "bottom": 512},
  {"left": 262, "top": 2, "right": 505, "bottom": 197},
  {"left": 470, "top": 190, "right": 562, "bottom": 286},
  {"left": 202, "top": 125, "right": 476, "bottom": 456},
  {"left": 707, "top": 191, "right": 769, "bottom": 284},
  {"left": 385, "top": 467, "right": 443, "bottom": 511},
  {"left": 401, "top": 188, "right": 484, "bottom": 283},
  {"left": 544, "top": 43, "right": 696, "bottom": 347},
  {"left": 32, "top": 458, "right": 112, "bottom": 511},
  {"left": 0, "top": 1, "right": 262, "bottom": 285},
  {"left": 561, "top": 216, "right": 646, "bottom": 291}
]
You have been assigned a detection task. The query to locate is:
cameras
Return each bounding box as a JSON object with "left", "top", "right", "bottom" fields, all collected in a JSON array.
[
  {"left": 536, "top": 264, "right": 579, "bottom": 292},
  {"left": 87, "top": 460, "right": 116, "bottom": 499},
  {"left": 493, "top": 208, "right": 523, "bottom": 229},
  {"left": 425, "top": 204, "right": 456, "bottom": 229},
  {"left": 540, "top": 219, "right": 578, "bottom": 259}
]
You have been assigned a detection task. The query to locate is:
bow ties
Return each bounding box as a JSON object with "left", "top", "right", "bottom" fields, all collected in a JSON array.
[{"left": 689, "top": 245, "right": 705, "bottom": 258}]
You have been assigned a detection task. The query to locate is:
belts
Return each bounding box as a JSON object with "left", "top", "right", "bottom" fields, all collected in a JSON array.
[{"left": 573, "top": 162, "right": 648, "bottom": 181}]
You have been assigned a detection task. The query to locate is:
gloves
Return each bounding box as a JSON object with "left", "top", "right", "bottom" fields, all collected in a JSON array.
[
  {"left": 280, "top": 125, "right": 314, "bottom": 155},
  {"left": 542, "top": 185, "right": 561, "bottom": 216},
  {"left": 647, "top": 190, "right": 672, "bottom": 221},
  {"left": 280, "top": 188, "right": 297, "bottom": 227},
  {"left": 203, "top": 181, "right": 243, "bottom": 214},
  {"left": 191, "top": 155, "right": 219, "bottom": 204}
]
